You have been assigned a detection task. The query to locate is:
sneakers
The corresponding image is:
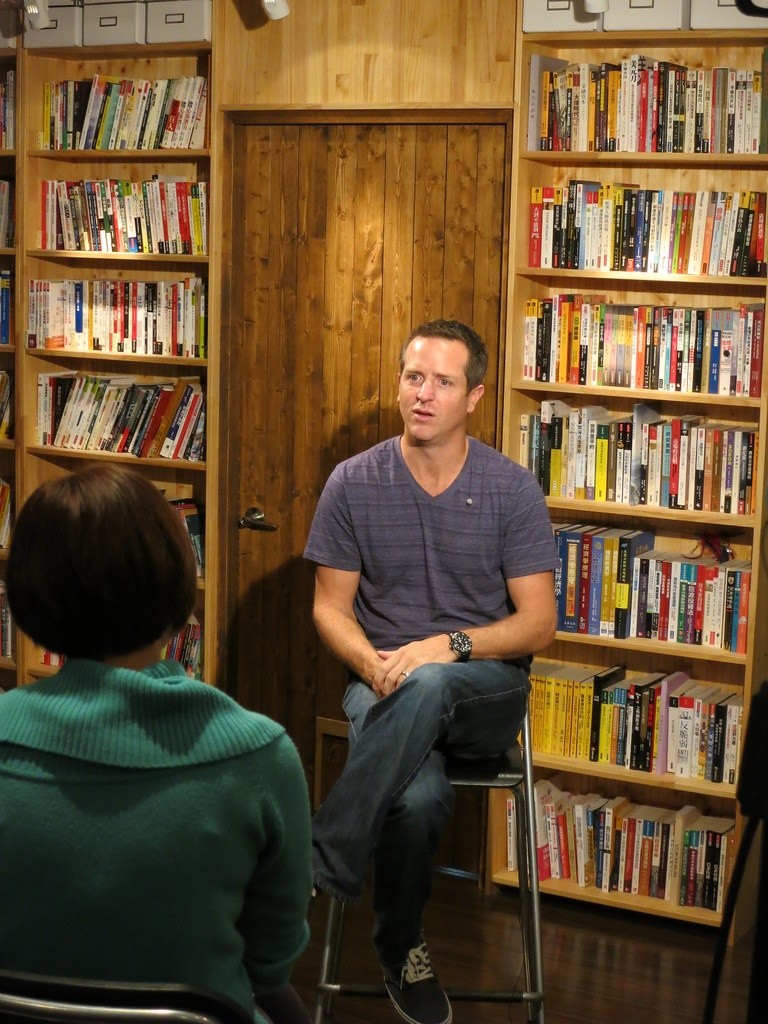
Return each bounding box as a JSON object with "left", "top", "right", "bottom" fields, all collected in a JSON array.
[{"left": 380, "top": 926, "right": 454, "bottom": 1024}]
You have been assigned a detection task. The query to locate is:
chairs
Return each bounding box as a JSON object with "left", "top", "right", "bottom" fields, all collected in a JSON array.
[
  {"left": 313, "top": 700, "right": 549, "bottom": 1024},
  {"left": 0, "top": 962, "right": 253, "bottom": 1024}
]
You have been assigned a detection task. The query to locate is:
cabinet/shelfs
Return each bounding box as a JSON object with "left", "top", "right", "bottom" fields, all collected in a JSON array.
[
  {"left": 0, "top": 41, "right": 235, "bottom": 701},
  {"left": 491, "top": 33, "right": 765, "bottom": 927}
]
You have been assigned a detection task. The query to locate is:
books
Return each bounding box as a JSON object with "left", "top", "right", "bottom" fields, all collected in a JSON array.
[
  {"left": 0, "top": 585, "right": 15, "bottom": 661},
  {"left": 529, "top": 663, "right": 744, "bottom": 786},
  {"left": 0, "top": 478, "right": 15, "bottom": 549},
  {"left": 519, "top": 399, "right": 757, "bottom": 515},
  {"left": 557, "top": 522, "right": 750, "bottom": 657},
  {"left": 161, "top": 620, "right": 204, "bottom": 682},
  {"left": 521, "top": 296, "right": 763, "bottom": 399},
  {"left": 0, "top": 69, "right": 15, "bottom": 150},
  {"left": 1, "top": 367, "right": 14, "bottom": 442},
  {"left": 33, "top": 643, "right": 68, "bottom": 670},
  {"left": 529, "top": 53, "right": 762, "bottom": 152},
  {"left": 27, "top": 278, "right": 209, "bottom": 359},
  {"left": 43, "top": 75, "right": 207, "bottom": 150},
  {"left": 506, "top": 775, "right": 734, "bottom": 913},
  {"left": 0, "top": 178, "right": 15, "bottom": 249},
  {"left": 42, "top": 175, "right": 209, "bottom": 255},
  {"left": 167, "top": 497, "right": 207, "bottom": 582},
  {"left": 0, "top": 270, "right": 14, "bottom": 345},
  {"left": 38, "top": 372, "right": 209, "bottom": 462},
  {"left": 529, "top": 183, "right": 766, "bottom": 278}
]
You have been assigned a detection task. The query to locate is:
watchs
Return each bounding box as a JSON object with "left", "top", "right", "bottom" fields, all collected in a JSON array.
[{"left": 445, "top": 630, "right": 474, "bottom": 661}]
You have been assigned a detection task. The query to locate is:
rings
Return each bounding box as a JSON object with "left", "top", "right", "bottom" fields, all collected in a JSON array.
[{"left": 401, "top": 670, "right": 410, "bottom": 677}]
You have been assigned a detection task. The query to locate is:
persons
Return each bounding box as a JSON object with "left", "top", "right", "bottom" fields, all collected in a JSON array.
[
  {"left": 0, "top": 465, "right": 316, "bottom": 1024},
  {"left": 303, "top": 321, "right": 558, "bottom": 1024}
]
